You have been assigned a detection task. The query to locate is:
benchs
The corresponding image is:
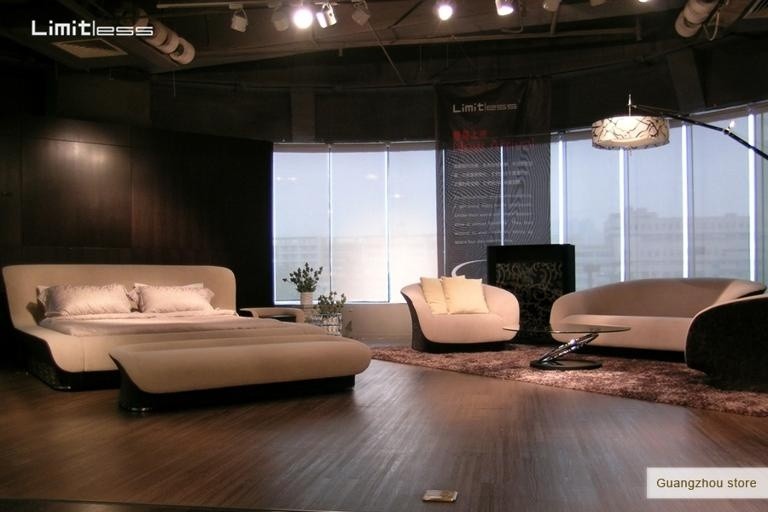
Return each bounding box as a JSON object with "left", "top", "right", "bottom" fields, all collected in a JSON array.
[{"left": 108, "top": 333, "right": 372, "bottom": 412}]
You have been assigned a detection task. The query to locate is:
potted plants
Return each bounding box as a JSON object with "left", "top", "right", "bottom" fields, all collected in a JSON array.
[{"left": 281, "top": 262, "right": 352, "bottom": 336}]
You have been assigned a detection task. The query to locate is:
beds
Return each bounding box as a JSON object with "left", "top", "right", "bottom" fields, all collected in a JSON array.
[{"left": 0, "top": 263, "right": 316, "bottom": 393}]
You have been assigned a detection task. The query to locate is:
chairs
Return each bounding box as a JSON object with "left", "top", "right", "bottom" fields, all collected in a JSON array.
[
  {"left": 683, "top": 290, "right": 766, "bottom": 391},
  {"left": 399, "top": 284, "right": 520, "bottom": 353}
]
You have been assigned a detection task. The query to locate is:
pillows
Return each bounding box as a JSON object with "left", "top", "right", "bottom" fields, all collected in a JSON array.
[
  {"left": 419, "top": 275, "right": 489, "bottom": 315},
  {"left": 36, "top": 281, "right": 217, "bottom": 317}
]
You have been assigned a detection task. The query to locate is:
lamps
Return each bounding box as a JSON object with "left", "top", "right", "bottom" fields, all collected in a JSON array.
[
  {"left": 432, "top": 0, "right": 561, "bottom": 19},
  {"left": 590, "top": 94, "right": 767, "bottom": 160},
  {"left": 228, "top": 0, "right": 370, "bottom": 32}
]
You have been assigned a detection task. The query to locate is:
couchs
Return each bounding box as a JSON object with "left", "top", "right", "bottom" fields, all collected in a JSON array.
[{"left": 549, "top": 277, "right": 765, "bottom": 368}]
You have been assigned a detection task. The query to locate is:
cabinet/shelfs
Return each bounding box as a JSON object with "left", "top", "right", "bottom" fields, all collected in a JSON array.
[{"left": 486, "top": 244, "right": 574, "bottom": 344}]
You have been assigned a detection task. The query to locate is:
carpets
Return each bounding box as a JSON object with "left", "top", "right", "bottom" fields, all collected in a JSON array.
[{"left": 370, "top": 344, "right": 767, "bottom": 417}]
